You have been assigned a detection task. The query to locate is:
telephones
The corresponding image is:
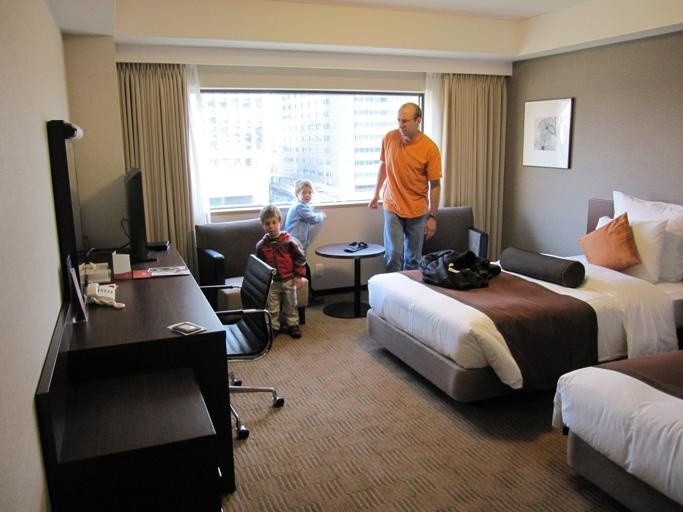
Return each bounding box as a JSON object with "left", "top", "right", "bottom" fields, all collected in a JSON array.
[{"left": 85, "top": 283, "right": 125, "bottom": 310}]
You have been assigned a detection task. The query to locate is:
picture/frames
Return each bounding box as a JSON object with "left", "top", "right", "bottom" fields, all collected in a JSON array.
[{"left": 522, "top": 97, "right": 574, "bottom": 169}]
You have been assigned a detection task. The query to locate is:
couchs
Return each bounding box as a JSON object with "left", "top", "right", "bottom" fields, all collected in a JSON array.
[
  {"left": 195, "top": 219, "right": 312, "bottom": 325},
  {"left": 422, "top": 206, "right": 489, "bottom": 259}
]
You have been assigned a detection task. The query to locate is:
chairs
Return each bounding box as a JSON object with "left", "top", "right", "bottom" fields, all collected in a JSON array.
[{"left": 199, "top": 254, "right": 285, "bottom": 440}]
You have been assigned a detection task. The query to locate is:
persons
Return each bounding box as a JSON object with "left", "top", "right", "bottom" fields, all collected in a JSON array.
[
  {"left": 254, "top": 205, "right": 306, "bottom": 339},
  {"left": 367, "top": 103, "right": 442, "bottom": 271},
  {"left": 283, "top": 178, "right": 326, "bottom": 307}
]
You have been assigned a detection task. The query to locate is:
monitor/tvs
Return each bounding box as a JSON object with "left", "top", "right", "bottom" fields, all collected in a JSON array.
[{"left": 124, "top": 167, "right": 157, "bottom": 262}]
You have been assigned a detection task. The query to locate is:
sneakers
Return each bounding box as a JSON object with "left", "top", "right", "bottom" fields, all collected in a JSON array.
[
  {"left": 288, "top": 325, "right": 300, "bottom": 339},
  {"left": 270, "top": 330, "right": 278, "bottom": 340},
  {"left": 311, "top": 297, "right": 325, "bottom": 305}
]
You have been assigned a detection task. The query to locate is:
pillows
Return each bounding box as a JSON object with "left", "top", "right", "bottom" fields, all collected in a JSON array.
[
  {"left": 500, "top": 246, "right": 585, "bottom": 289},
  {"left": 595, "top": 215, "right": 668, "bottom": 283},
  {"left": 578, "top": 212, "right": 641, "bottom": 271},
  {"left": 612, "top": 190, "right": 683, "bottom": 282}
]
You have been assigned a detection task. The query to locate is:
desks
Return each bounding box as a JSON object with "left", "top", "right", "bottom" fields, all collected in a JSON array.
[
  {"left": 315, "top": 242, "right": 385, "bottom": 319},
  {"left": 35, "top": 241, "right": 236, "bottom": 512}
]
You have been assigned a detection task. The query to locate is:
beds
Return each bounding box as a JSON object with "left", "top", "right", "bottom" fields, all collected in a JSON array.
[
  {"left": 366, "top": 200, "right": 683, "bottom": 402},
  {"left": 551, "top": 352, "right": 682, "bottom": 511}
]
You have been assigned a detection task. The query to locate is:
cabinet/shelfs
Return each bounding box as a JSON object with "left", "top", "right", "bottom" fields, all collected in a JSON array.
[{"left": 59, "top": 365, "right": 224, "bottom": 512}]
